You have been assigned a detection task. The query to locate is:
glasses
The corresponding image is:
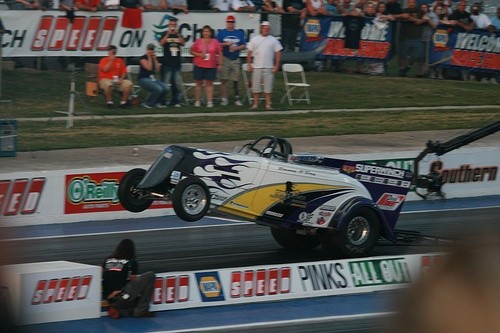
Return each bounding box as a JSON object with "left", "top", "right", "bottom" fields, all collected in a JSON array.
[{"left": 227, "top": 21, "right": 235, "bottom": 23}]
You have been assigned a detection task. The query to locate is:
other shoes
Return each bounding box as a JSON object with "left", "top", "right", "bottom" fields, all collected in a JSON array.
[
  {"left": 250, "top": 105, "right": 258, "bottom": 109},
  {"left": 141, "top": 103, "right": 152, "bottom": 109},
  {"left": 155, "top": 103, "right": 168, "bottom": 109},
  {"left": 134, "top": 311, "right": 156, "bottom": 317},
  {"left": 106, "top": 104, "right": 115, "bottom": 109},
  {"left": 235, "top": 101, "right": 242, "bottom": 106},
  {"left": 206, "top": 101, "right": 214, "bottom": 107},
  {"left": 266, "top": 105, "right": 272, "bottom": 110},
  {"left": 194, "top": 101, "right": 201, "bottom": 107},
  {"left": 119, "top": 103, "right": 130, "bottom": 109},
  {"left": 108, "top": 306, "right": 120, "bottom": 319},
  {"left": 173, "top": 104, "right": 182, "bottom": 108},
  {"left": 220, "top": 100, "right": 229, "bottom": 106}
]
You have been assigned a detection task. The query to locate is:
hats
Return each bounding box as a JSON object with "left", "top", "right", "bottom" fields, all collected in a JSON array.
[
  {"left": 226, "top": 16, "right": 234, "bottom": 21},
  {"left": 260, "top": 21, "right": 270, "bottom": 26},
  {"left": 147, "top": 43, "right": 156, "bottom": 49}
]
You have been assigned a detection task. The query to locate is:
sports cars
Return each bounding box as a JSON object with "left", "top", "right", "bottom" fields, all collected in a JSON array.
[{"left": 116, "top": 134, "right": 421, "bottom": 258}]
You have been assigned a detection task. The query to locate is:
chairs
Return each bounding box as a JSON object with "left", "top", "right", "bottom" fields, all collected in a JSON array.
[
  {"left": 84, "top": 62, "right": 220, "bottom": 105},
  {"left": 242, "top": 63, "right": 274, "bottom": 105},
  {"left": 280, "top": 62, "right": 311, "bottom": 106}
]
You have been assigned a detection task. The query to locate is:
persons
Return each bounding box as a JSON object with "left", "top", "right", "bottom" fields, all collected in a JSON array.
[
  {"left": 161, "top": 18, "right": 186, "bottom": 108},
  {"left": 98, "top": 45, "right": 132, "bottom": 108},
  {"left": 247, "top": 21, "right": 283, "bottom": 109},
  {"left": 190, "top": 25, "right": 223, "bottom": 108},
  {"left": 103, "top": 239, "right": 157, "bottom": 319},
  {"left": 218, "top": 15, "right": 246, "bottom": 107},
  {"left": 137, "top": 44, "right": 168, "bottom": 108},
  {"left": 4, "top": 0, "right": 500, "bottom": 84}
]
